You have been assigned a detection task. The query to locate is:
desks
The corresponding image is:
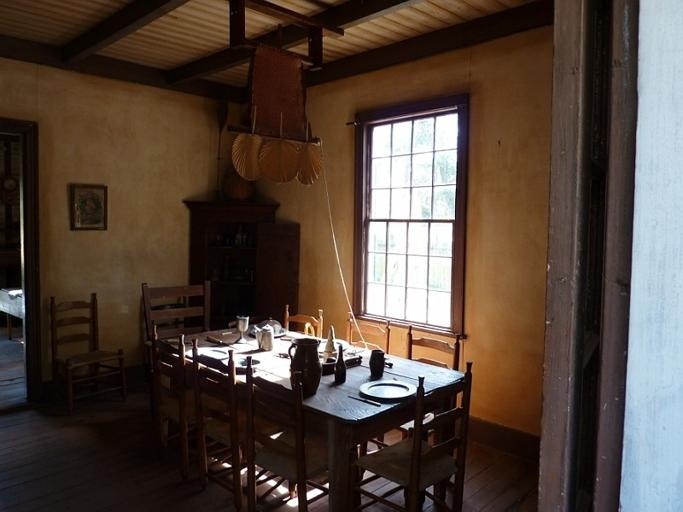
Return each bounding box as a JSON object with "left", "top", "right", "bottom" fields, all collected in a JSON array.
[{"left": 0, "top": 288, "right": 23, "bottom": 340}]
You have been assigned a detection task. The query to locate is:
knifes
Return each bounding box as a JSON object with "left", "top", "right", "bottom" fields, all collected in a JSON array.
[
  {"left": 346, "top": 393, "right": 380, "bottom": 406},
  {"left": 207, "top": 336, "right": 237, "bottom": 350}
]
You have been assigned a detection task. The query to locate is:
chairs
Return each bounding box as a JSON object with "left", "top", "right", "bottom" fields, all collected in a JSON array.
[
  {"left": 138, "top": 281, "right": 473, "bottom": 512},
  {"left": 50, "top": 293, "right": 127, "bottom": 413}
]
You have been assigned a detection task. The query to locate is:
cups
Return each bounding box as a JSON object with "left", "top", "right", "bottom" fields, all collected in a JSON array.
[{"left": 367, "top": 348, "right": 386, "bottom": 376}]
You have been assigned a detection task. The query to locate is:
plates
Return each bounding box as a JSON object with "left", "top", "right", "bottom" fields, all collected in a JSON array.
[
  {"left": 357, "top": 380, "right": 417, "bottom": 402},
  {"left": 251, "top": 326, "right": 285, "bottom": 337},
  {"left": 314, "top": 336, "right": 351, "bottom": 354},
  {"left": 223, "top": 358, "right": 262, "bottom": 372},
  {"left": 188, "top": 347, "right": 233, "bottom": 363}
]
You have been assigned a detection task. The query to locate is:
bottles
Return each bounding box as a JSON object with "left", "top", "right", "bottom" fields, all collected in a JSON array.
[{"left": 334, "top": 345, "right": 346, "bottom": 382}]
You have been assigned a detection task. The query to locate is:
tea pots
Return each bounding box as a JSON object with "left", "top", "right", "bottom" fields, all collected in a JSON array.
[
  {"left": 253, "top": 324, "right": 274, "bottom": 350},
  {"left": 286, "top": 337, "right": 323, "bottom": 400}
]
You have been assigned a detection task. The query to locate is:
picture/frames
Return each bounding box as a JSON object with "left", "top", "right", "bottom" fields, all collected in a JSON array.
[{"left": 69, "top": 183, "right": 108, "bottom": 232}]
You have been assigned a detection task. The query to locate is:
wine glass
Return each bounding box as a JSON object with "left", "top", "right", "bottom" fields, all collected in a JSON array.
[{"left": 235, "top": 315, "right": 250, "bottom": 344}]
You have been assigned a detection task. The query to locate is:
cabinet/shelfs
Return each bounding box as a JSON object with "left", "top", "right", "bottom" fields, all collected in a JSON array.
[{"left": 181, "top": 199, "right": 300, "bottom": 328}]
